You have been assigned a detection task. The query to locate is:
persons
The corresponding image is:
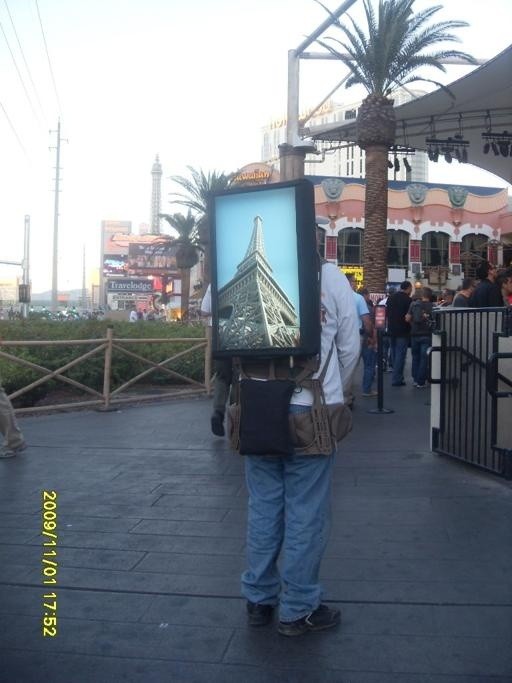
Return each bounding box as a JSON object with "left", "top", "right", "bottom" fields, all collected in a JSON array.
[
  {"left": 240, "top": 227, "right": 364, "bottom": 635},
  {"left": 0, "top": 388, "right": 28, "bottom": 458},
  {"left": 201, "top": 280, "right": 238, "bottom": 436},
  {"left": 129, "top": 307, "right": 166, "bottom": 324},
  {"left": 1, "top": 303, "right": 105, "bottom": 321},
  {"left": 439, "top": 259, "right": 512, "bottom": 306},
  {"left": 344, "top": 272, "right": 435, "bottom": 410}
]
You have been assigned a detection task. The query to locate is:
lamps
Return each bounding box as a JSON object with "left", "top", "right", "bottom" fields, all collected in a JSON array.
[{"left": 388, "top": 108, "right": 512, "bottom": 172}]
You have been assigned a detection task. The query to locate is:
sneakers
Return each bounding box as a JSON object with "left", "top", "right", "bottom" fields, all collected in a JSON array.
[
  {"left": 278, "top": 603, "right": 342, "bottom": 637},
  {"left": 0, "top": 435, "right": 27, "bottom": 459},
  {"left": 361, "top": 390, "right": 378, "bottom": 399},
  {"left": 391, "top": 380, "right": 405, "bottom": 387},
  {"left": 413, "top": 381, "right": 426, "bottom": 389},
  {"left": 244, "top": 599, "right": 276, "bottom": 628},
  {"left": 210, "top": 410, "right": 226, "bottom": 438}
]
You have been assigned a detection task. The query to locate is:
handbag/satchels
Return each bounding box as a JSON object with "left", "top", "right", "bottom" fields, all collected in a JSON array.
[{"left": 237, "top": 375, "right": 299, "bottom": 457}]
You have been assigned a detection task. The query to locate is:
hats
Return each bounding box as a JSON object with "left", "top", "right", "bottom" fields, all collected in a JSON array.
[{"left": 415, "top": 287, "right": 433, "bottom": 299}]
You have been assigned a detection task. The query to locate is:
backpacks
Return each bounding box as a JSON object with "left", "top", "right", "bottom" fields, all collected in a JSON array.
[{"left": 414, "top": 308, "right": 435, "bottom": 330}]
